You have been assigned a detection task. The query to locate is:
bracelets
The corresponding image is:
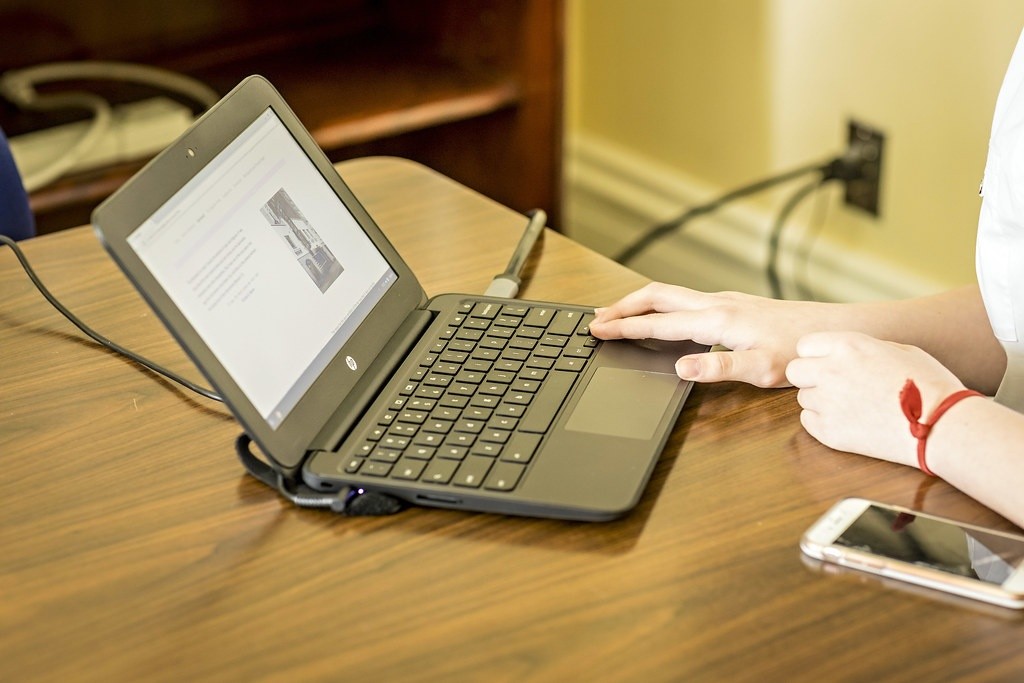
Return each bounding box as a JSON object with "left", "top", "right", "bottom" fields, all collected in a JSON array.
[
  {"left": 891, "top": 475, "right": 940, "bottom": 530},
  {"left": 900, "top": 379, "right": 986, "bottom": 477}
]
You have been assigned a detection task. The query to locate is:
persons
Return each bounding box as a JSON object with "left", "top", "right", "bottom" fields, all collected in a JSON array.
[
  {"left": 589, "top": 28, "right": 1024, "bottom": 530},
  {"left": 784, "top": 429, "right": 1011, "bottom": 534}
]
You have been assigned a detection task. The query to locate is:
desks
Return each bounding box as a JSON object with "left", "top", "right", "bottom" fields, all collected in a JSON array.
[{"left": 0, "top": 157, "right": 1024, "bottom": 683}]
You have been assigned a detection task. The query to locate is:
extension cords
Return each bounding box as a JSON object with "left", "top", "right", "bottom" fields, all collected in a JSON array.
[{"left": 9, "top": 97, "right": 186, "bottom": 193}]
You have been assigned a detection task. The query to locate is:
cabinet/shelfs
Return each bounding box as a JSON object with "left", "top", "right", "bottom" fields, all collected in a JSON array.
[{"left": 0, "top": 0, "right": 565, "bottom": 234}]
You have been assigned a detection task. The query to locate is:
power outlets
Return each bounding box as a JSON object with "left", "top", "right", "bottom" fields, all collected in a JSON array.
[{"left": 842, "top": 122, "right": 883, "bottom": 214}]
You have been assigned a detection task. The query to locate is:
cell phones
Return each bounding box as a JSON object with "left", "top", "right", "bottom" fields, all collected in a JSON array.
[{"left": 799, "top": 496, "right": 1024, "bottom": 612}]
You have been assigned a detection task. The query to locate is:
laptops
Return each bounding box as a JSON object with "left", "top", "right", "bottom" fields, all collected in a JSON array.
[{"left": 91, "top": 74, "right": 712, "bottom": 522}]
